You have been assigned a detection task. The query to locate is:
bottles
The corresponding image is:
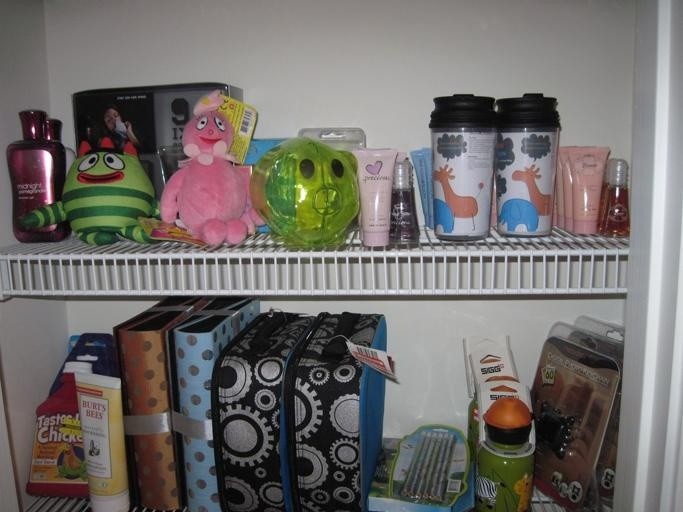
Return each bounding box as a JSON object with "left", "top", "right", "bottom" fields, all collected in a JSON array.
[{"left": 474, "top": 395, "right": 538, "bottom": 511}]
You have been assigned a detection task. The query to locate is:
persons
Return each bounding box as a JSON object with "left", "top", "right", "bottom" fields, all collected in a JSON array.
[{"left": 102, "top": 107, "right": 141, "bottom": 149}]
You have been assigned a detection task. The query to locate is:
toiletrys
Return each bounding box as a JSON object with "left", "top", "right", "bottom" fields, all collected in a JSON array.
[
  {"left": 597, "top": 157, "right": 630, "bottom": 237},
  {"left": 389, "top": 162, "right": 420, "bottom": 242}
]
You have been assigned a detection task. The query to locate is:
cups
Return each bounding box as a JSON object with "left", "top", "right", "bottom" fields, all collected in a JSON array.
[
  {"left": 428, "top": 92, "right": 499, "bottom": 240},
  {"left": 494, "top": 92, "right": 562, "bottom": 239}
]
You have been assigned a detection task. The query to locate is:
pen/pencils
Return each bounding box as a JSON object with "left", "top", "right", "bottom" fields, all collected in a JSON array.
[{"left": 400, "top": 431, "right": 456, "bottom": 501}]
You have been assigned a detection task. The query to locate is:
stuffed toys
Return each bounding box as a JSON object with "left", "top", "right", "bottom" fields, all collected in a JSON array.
[
  {"left": 21, "top": 137, "right": 158, "bottom": 245},
  {"left": 160, "top": 91, "right": 266, "bottom": 249}
]
